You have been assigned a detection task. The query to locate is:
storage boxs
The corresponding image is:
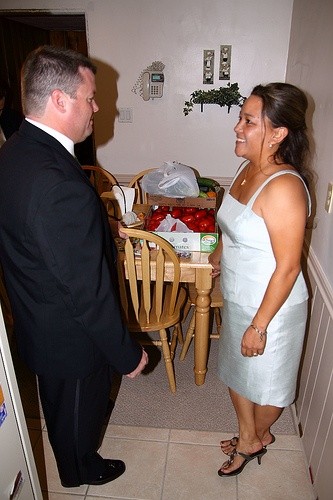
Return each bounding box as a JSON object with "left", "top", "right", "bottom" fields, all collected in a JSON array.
[
  {"left": 148, "top": 189, "right": 216, "bottom": 206},
  {"left": 140, "top": 205, "right": 218, "bottom": 253}
]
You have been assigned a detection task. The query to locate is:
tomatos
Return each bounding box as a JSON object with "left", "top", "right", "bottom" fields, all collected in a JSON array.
[{"left": 146, "top": 206, "right": 216, "bottom": 233}]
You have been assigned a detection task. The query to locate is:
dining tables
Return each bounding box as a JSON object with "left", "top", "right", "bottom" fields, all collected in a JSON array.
[{"left": 103, "top": 204, "right": 216, "bottom": 386}]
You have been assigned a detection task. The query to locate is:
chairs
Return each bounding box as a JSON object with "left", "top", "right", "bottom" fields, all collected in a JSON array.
[{"left": 80, "top": 166, "right": 224, "bottom": 394}]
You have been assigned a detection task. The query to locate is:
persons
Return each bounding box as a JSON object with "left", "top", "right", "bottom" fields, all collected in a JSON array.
[
  {"left": 208, "top": 82, "right": 318, "bottom": 477},
  {"left": 0, "top": 44, "right": 149, "bottom": 488}
]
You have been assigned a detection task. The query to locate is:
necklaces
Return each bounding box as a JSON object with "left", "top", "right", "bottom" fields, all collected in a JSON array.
[{"left": 240, "top": 163, "right": 270, "bottom": 185}]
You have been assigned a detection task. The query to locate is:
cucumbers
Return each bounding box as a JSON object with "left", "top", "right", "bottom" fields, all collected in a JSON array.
[{"left": 196, "top": 177, "right": 216, "bottom": 187}]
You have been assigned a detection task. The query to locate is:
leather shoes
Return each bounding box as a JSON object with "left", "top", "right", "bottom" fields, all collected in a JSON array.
[{"left": 62, "top": 459, "right": 125, "bottom": 487}]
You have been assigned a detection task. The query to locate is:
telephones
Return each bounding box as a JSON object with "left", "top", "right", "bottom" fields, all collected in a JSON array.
[{"left": 142, "top": 70, "right": 165, "bottom": 101}]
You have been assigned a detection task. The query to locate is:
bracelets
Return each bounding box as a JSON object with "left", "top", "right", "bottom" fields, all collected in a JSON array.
[{"left": 250, "top": 323, "right": 268, "bottom": 343}]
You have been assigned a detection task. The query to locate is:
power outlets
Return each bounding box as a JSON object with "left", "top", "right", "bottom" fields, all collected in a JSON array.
[{"left": 325, "top": 182, "right": 333, "bottom": 213}]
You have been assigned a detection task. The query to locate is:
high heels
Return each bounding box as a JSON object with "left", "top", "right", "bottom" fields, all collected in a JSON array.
[
  {"left": 218, "top": 448, "right": 267, "bottom": 477},
  {"left": 220, "top": 432, "right": 275, "bottom": 456}
]
